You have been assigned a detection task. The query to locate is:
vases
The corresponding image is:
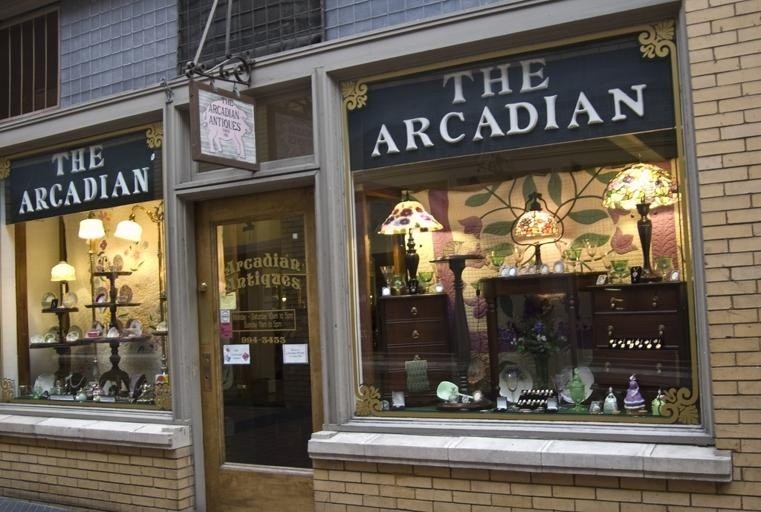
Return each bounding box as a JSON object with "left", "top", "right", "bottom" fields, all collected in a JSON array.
[{"left": 532, "top": 354, "right": 551, "bottom": 390}]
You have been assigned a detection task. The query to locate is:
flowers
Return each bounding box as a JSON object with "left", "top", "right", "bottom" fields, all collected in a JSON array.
[{"left": 497, "top": 293, "right": 571, "bottom": 360}]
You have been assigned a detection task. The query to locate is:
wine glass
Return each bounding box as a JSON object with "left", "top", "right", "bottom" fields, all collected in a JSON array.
[
  {"left": 565, "top": 368, "right": 587, "bottom": 412},
  {"left": 490, "top": 251, "right": 505, "bottom": 273},
  {"left": 419, "top": 270, "right": 433, "bottom": 292},
  {"left": 565, "top": 239, "right": 598, "bottom": 275},
  {"left": 655, "top": 256, "right": 673, "bottom": 281},
  {"left": 504, "top": 369, "right": 519, "bottom": 409},
  {"left": 381, "top": 265, "right": 395, "bottom": 287},
  {"left": 599, "top": 252, "right": 627, "bottom": 284}
]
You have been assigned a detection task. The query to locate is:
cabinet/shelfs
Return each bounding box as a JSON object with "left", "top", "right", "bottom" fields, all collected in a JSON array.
[
  {"left": 374, "top": 293, "right": 451, "bottom": 408},
  {"left": 579, "top": 280, "right": 688, "bottom": 410}
]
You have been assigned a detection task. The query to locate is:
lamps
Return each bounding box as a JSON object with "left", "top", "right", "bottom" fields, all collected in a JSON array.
[
  {"left": 378, "top": 200, "right": 444, "bottom": 294},
  {"left": 602, "top": 161, "right": 682, "bottom": 282},
  {"left": 510, "top": 191, "right": 564, "bottom": 273},
  {"left": 51, "top": 201, "right": 168, "bottom": 374}
]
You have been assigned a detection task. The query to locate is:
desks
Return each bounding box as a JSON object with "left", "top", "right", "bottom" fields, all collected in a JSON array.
[{"left": 479, "top": 273, "right": 609, "bottom": 405}]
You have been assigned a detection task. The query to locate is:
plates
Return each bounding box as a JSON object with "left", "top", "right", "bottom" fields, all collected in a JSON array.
[
  {"left": 559, "top": 365, "right": 596, "bottom": 403},
  {"left": 41, "top": 286, "right": 143, "bottom": 342},
  {"left": 154, "top": 320, "right": 169, "bottom": 331}
]
